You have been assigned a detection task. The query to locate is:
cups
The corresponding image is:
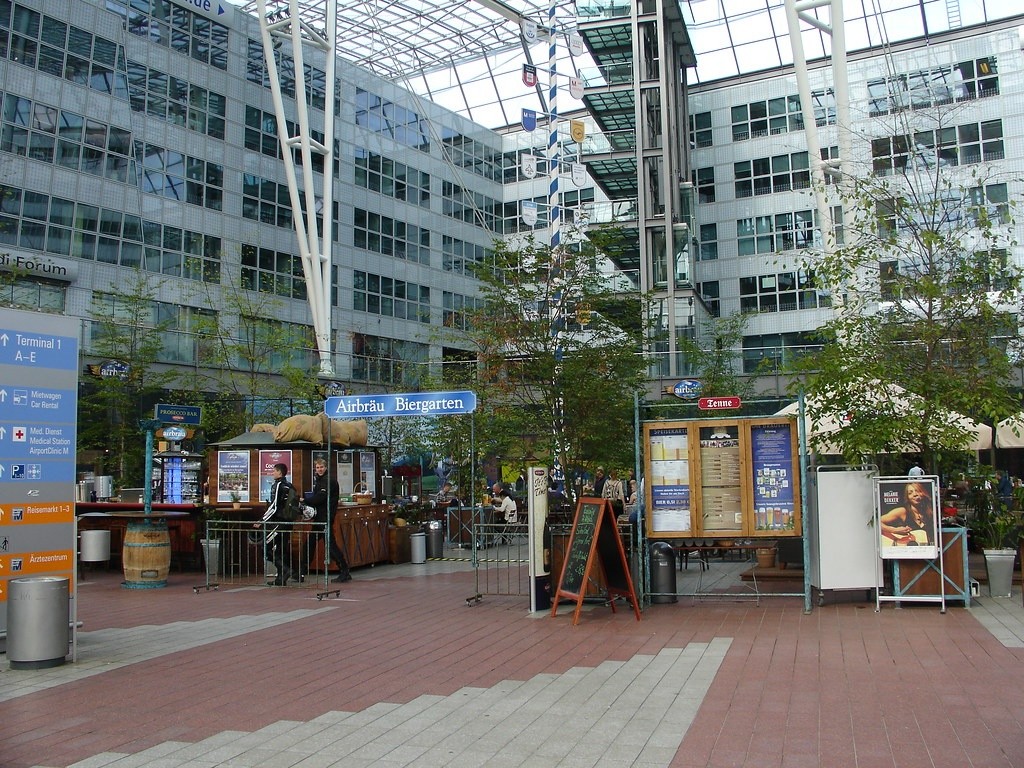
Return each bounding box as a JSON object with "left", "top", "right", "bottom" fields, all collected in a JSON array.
[{"left": 754, "top": 506, "right": 794, "bottom": 529}]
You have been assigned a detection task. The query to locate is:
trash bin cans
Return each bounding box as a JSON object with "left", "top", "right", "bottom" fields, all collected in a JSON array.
[
  {"left": 76, "top": 483, "right": 86, "bottom": 502},
  {"left": 410, "top": 533, "right": 427, "bottom": 564},
  {"left": 426, "top": 520, "right": 444, "bottom": 558},
  {"left": 649, "top": 542, "right": 677, "bottom": 604}
]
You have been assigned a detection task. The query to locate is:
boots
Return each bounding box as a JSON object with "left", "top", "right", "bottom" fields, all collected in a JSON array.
[
  {"left": 292, "top": 561, "right": 312, "bottom": 582},
  {"left": 331, "top": 559, "right": 352, "bottom": 582}
]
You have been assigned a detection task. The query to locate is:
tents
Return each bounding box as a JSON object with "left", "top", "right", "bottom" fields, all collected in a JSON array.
[
  {"left": 773, "top": 376, "right": 992, "bottom": 455},
  {"left": 995, "top": 410, "right": 1024, "bottom": 448}
]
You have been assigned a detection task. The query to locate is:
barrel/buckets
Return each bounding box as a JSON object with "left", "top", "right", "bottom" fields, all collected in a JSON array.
[
  {"left": 123, "top": 523, "right": 171, "bottom": 585},
  {"left": 756, "top": 548, "right": 776, "bottom": 569}
]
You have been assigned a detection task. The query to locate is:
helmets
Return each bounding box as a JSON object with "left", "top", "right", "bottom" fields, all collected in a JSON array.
[
  {"left": 301, "top": 504, "right": 317, "bottom": 520},
  {"left": 248, "top": 527, "right": 264, "bottom": 543}
]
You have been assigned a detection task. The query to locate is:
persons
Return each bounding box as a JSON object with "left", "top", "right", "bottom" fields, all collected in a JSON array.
[
  {"left": 292, "top": 457, "right": 352, "bottom": 583},
  {"left": 253, "top": 463, "right": 297, "bottom": 586},
  {"left": 880, "top": 482, "right": 934, "bottom": 544},
  {"left": 434, "top": 483, "right": 453, "bottom": 514},
  {"left": 955, "top": 474, "right": 975, "bottom": 511},
  {"left": 908, "top": 462, "right": 925, "bottom": 480},
  {"left": 481, "top": 467, "right": 645, "bottom": 541},
  {"left": 995, "top": 471, "right": 1012, "bottom": 509}
]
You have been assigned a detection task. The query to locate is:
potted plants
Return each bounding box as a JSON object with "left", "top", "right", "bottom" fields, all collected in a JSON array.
[
  {"left": 190, "top": 506, "right": 229, "bottom": 575},
  {"left": 761, "top": 75, "right": 1024, "bottom": 609},
  {"left": 393, "top": 501, "right": 409, "bottom": 527},
  {"left": 448, "top": 201, "right": 677, "bottom": 604},
  {"left": 220, "top": 474, "right": 247, "bottom": 509},
  {"left": 961, "top": 464, "right": 1024, "bottom": 598}
]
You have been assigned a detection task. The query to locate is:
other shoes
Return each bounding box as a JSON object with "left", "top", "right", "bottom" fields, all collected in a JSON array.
[
  {"left": 277, "top": 571, "right": 291, "bottom": 585},
  {"left": 267, "top": 580, "right": 286, "bottom": 586}
]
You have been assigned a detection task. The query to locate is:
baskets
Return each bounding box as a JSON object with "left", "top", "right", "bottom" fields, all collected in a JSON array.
[{"left": 351, "top": 482, "right": 373, "bottom": 504}]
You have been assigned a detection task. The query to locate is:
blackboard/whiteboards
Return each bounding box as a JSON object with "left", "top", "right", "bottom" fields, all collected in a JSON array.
[{"left": 551, "top": 497, "right": 642, "bottom": 600}]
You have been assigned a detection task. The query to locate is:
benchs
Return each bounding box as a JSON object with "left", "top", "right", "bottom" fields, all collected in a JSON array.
[{"left": 672, "top": 540, "right": 777, "bottom": 571}]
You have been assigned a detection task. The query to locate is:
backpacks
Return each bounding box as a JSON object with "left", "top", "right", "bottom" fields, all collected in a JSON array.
[
  {"left": 280, "top": 488, "right": 300, "bottom": 520},
  {"left": 604, "top": 480, "right": 619, "bottom": 501}
]
